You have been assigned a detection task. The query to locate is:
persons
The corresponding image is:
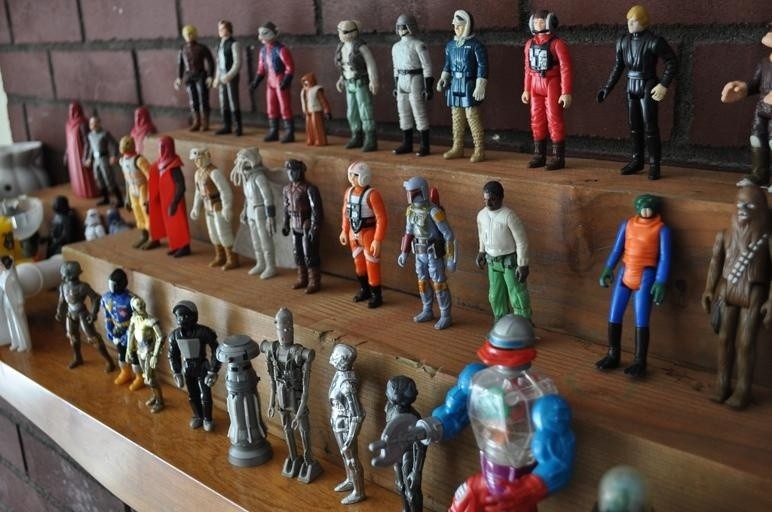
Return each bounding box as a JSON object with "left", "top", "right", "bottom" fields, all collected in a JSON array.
[
  {"left": 173, "top": 25, "right": 215, "bottom": 132},
  {"left": 596, "top": 5, "right": 678, "bottom": 181},
  {"left": 391, "top": 13, "right": 435, "bottom": 157},
  {"left": 334, "top": 19, "right": 380, "bottom": 153},
  {"left": 300, "top": 73, "right": 332, "bottom": 146},
  {"left": 60, "top": 100, "right": 771, "bottom": 409},
  {"left": 249, "top": 21, "right": 295, "bottom": 143},
  {"left": 49, "top": 199, "right": 127, "bottom": 255},
  {"left": 1, "top": 258, "right": 659, "bottom": 510},
  {"left": 720, "top": 22, "right": 772, "bottom": 193},
  {"left": 435, "top": 9, "right": 488, "bottom": 163},
  {"left": 212, "top": 19, "right": 242, "bottom": 137},
  {"left": 0, "top": 100, "right": 771, "bottom": 512},
  {"left": 521, "top": 11, "right": 574, "bottom": 171}
]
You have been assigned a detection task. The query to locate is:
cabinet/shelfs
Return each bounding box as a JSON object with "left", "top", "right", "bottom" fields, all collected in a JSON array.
[{"left": 0, "top": 126, "right": 771, "bottom": 512}]
